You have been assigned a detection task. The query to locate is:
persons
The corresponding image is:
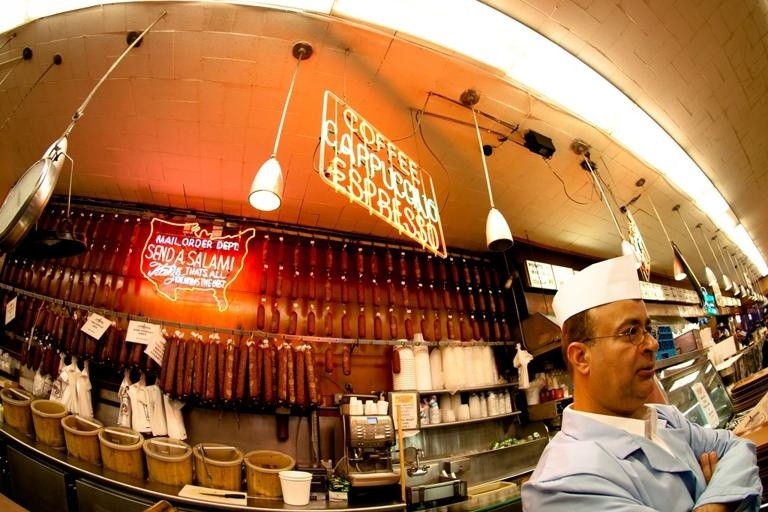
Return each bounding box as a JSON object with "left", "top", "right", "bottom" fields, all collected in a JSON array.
[
  {"left": 520, "top": 254, "right": 763, "bottom": 510},
  {"left": 736, "top": 328, "right": 749, "bottom": 347}
]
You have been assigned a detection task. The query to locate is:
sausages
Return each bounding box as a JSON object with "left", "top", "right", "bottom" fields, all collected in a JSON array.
[
  {"left": 325, "top": 309, "right": 509, "bottom": 342},
  {"left": 325, "top": 348, "right": 334, "bottom": 373},
  {"left": 258, "top": 268, "right": 506, "bottom": 312},
  {"left": 0, "top": 291, "right": 323, "bottom": 405},
  {"left": 1, "top": 218, "right": 142, "bottom": 312},
  {"left": 342, "top": 345, "right": 351, "bottom": 376},
  {"left": 261, "top": 238, "right": 501, "bottom": 288}
]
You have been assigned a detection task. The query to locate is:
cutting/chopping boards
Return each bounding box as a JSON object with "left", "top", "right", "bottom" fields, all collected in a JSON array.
[{"left": 178, "top": 483, "right": 248, "bottom": 508}]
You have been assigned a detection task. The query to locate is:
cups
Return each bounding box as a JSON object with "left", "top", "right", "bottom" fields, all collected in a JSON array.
[
  {"left": 348, "top": 396, "right": 388, "bottom": 416},
  {"left": 390, "top": 333, "right": 500, "bottom": 423},
  {"left": 276, "top": 469, "right": 314, "bottom": 507}
]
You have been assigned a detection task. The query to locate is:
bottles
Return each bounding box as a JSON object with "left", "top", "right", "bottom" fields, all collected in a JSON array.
[{"left": 468, "top": 389, "right": 513, "bottom": 421}]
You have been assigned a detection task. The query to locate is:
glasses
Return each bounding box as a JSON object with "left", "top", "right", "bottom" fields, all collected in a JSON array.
[{"left": 580, "top": 323, "right": 656, "bottom": 345}]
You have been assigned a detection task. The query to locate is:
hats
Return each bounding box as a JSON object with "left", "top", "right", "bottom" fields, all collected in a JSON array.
[{"left": 551, "top": 254, "right": 644, "bottom": 331}]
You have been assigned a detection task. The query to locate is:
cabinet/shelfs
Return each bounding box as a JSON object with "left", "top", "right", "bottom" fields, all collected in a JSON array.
[{"left": 392, "top": 323, "right": 766, "bottom": 511}]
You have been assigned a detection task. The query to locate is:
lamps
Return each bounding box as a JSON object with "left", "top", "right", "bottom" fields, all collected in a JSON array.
[
  {"left": 247, "top": 54, "right": 303, "bottom": 213},
  {"left": 46, "top": 165, "right": 88, "bottom": 257},
  {"left": 677, "top": 209, "right": 718, "bottom": 287},
  {"left": 641, "top": 184, "right": 688, "bottom": 282},
  {"left": 469, "top": 101, "right": 514, "bottom": 254},
  {"left": 582, "top": 152, "right": 643, "bottom": 270},
  {"left": 717, "top": 243, "right": 768, "bottom": 304},
  {"left": 701, "top": 227, "right": 733, "bottom": 291}
]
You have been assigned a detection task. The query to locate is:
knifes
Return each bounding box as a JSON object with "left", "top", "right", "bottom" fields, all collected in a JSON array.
[{"left": 198, "top": 492, "right": 245, "bottom": 499}]
